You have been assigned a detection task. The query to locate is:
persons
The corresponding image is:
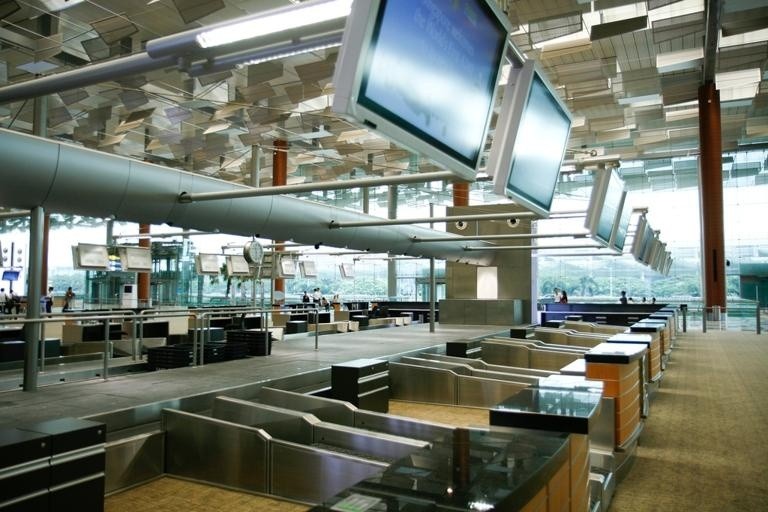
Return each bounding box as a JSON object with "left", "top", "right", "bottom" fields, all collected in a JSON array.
[
  {"left": 62, "top": 287, "right": 74, "bottom": 311},
  {"left": 553, "top": 288, "right": 562, "bottom": 303},
  {"left": 560, "top": 291, "right": 568, "bottom": 304},
  {"left": 303, "top": 287, "right": 340, "bottom": 312},
  {"left": 620, "top": 291, "right": 656, "bottom": 304},
  {"left": 0, "top": 288, "right": 16, "bottom": 314},
  {"left": 46, "top": 287, "right": 54, "bottom": 318}
]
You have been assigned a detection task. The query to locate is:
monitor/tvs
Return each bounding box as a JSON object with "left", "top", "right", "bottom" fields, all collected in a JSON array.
[
  {"left": 487, "top": 58, "right": 574, "bottom": 219},
  {"left": 631, "top": 215, "right": 674, "bottom": 278},
  {"left": 195, "top": 252, "right": 221, "bottom": 275},
  {"left": 71, "top": 241, "right": 113, "bottom": 273},
  {"left": 331, "top": 0, "right": 515, "bottom": 181},
  {"left": 299, "top": 261, "right": 318, "bottom": 278},
  {"left": 278, "top": 258, "right": 298, "bottom": 279},
  {"left": 226, "top": 255, "right": 254, "bottom": 276},
  {"left": 118, "top": 246, "right": 154, "bottom": 274},
  {"left": 611, "top": 191, "right": 629, "bottom": 253},
  {"left": 586, "top": 165, "right": 626, "bottom": 248},
  {"left": 339, "top": 263, "right": 355, "bottom": 279}
]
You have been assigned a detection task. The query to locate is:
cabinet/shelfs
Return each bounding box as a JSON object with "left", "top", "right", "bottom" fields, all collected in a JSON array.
[
  {"left": 446, "top": 338, "right": 483, "bottom": 361},
  {"left": 509, "top": 327, "right": 536, "bottom": 341},
  {"left": 330, "top": 355, "right": 390, "bottom": 415},
  {"left": 0, "top": 417, "right": 106, "bottom": 512}
]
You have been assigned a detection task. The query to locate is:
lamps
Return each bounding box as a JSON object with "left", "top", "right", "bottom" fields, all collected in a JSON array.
[{"left": 145, "top": 1, "right": 353, "bottom": 76}]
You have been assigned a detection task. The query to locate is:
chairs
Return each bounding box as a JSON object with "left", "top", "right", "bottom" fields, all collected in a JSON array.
[
  {"left": 1, "top": 303, "right": 271, "bottom": 369},
  {"left": 260, "top": 301, "right": 441, "bottom": 351}
]
